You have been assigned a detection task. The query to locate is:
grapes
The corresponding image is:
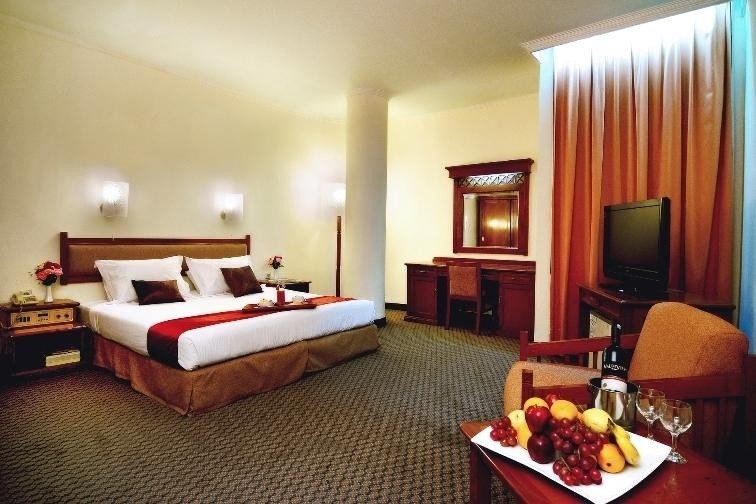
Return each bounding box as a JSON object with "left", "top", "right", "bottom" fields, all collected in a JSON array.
[
  {"left": 490, "top": 415, "right": 517, "bottom": 447},
  {"left": 548, "top": 418, "right": 608, "bottom": 486}
]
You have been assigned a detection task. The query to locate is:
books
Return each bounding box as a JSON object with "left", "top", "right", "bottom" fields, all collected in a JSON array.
[{"left": 39, "top": 346, "right": 80, "bottom": 368}]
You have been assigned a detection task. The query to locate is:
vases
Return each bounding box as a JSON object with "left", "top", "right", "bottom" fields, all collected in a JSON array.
[
  {"left": 43, "top": 285, "right": 53, "bottom": 303},
  {"left": 272, "top": 269, "right": 279, "bottom": 279}
]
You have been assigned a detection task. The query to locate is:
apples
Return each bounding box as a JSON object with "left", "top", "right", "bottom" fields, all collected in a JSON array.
[{"left": 508, "top": 397, "right": 578, "bottom": 464}]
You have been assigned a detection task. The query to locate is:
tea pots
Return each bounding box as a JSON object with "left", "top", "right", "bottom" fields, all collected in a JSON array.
[
  {"left": 292, "top": 295, "right": 305, "bottom": 305},
  {"left": 256, "top": 298, "right": 275, "bottom": 307}
]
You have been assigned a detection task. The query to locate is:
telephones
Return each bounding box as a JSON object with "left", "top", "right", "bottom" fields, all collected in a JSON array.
[{"left": 10, "top": 293, "right": 39, "bottom": 306}]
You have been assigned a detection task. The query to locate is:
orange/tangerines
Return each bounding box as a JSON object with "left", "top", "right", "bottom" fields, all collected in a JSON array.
[{"left": 582, "top": 408, "right": 613, "bottom": 433}]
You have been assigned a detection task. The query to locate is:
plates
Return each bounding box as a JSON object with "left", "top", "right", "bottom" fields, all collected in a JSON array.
[{"left": 467, "top": 420, "right": 673, "bottom": 503}]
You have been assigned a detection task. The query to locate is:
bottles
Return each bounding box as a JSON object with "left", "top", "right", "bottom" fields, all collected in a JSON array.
[{"left": 600, "top": 318, "right": 627, "bottom": 392}]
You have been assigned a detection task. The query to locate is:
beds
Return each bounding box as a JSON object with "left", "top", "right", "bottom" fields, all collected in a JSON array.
[{"left": 53, "top": 231, "right": 382, "bottom": 418}]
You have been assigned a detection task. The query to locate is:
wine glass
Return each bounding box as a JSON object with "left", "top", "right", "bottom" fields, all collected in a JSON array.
[{"left": 636, "top": 387, "right": 693, "bottom": 465}]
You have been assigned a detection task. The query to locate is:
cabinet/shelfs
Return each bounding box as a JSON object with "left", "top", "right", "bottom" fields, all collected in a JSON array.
[{"left": 477, "top": 195, "right": 517, "bottom": 248}]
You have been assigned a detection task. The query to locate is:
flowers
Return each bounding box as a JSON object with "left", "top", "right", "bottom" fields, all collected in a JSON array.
[
  {"left": 26, "top": 261, "right": 63, "bottom": 286},
  {"left": 267, "top": 255, "right": 284, "bottom": 269}
]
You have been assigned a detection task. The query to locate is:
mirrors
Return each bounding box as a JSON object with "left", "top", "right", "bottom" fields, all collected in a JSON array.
[{"left": 443, "top": 157, "right": 535, "bottom": 256}]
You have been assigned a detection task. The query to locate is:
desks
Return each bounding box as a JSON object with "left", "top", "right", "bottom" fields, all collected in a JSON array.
[
  {"left": 457, "top": 418, "right": 755, "bottom": 503},
  {"left": 403, "top": 256, "right": 536, "bottom": 341},
  {"left": 573, "top": 281, "right": 736, "bottom": 368}
]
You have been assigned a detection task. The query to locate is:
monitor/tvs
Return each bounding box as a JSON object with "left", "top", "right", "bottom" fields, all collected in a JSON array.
[{"left": 599, "top": 197, "right": 670, "bottom": 297}]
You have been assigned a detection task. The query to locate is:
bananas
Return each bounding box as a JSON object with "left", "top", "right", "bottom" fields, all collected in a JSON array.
[{"left": 609, "top": 419, "right": 641, "bottom": 466}]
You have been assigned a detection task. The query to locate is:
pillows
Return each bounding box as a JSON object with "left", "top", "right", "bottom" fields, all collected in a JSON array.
[
  {"left": 218, "top": 266, "right": 264, "bottom": 298},
  {"left": 93, "top": 254, "right": 189, "bottom": 304},
  {"left": 183, "top": 254, "right": 254, "bottom": 297},
  {"left": 100, "top": 277, "right": 112, "bottom": 302},
  {"left": 184, "top": 268, "right": 199, "bottom": 294},
  {"left": 130, "top": 279, "right": 185, "bottom": 306}
]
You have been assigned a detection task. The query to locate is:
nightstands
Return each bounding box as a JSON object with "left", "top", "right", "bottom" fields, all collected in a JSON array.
[
  {"left": 0, "top": 321, "right": 94, "bottom": 386},
  {"left": 257, "top": 279, "right": 311, "bottom": 293}
]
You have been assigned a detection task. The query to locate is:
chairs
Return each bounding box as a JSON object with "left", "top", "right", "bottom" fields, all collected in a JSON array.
[
  {"left": 501, "top": 301, "right": 755, "bottom": 484},
  {"left": 444, "top": 260, "right": 495, "bottom": 337}
]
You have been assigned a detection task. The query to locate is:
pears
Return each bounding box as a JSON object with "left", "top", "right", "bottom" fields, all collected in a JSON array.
[{"left": 597, "top": 443, "right": 626, "bottom": 473}]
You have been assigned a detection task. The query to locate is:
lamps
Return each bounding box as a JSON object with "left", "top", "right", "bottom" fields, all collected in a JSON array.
[
  {"left": 97, "top": 181, "right": 128, "bottom": 218},
  {"left": 220, "top": 193, "right": 243, "bottom": 222}
]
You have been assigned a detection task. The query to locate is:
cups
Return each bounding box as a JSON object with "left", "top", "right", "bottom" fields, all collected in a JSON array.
[
  {"left": 276, "top": 290, "right": 285, "bottom": 305},
  {"left": 585, "top": 377, "right": 638, "bottom": 431}
]
